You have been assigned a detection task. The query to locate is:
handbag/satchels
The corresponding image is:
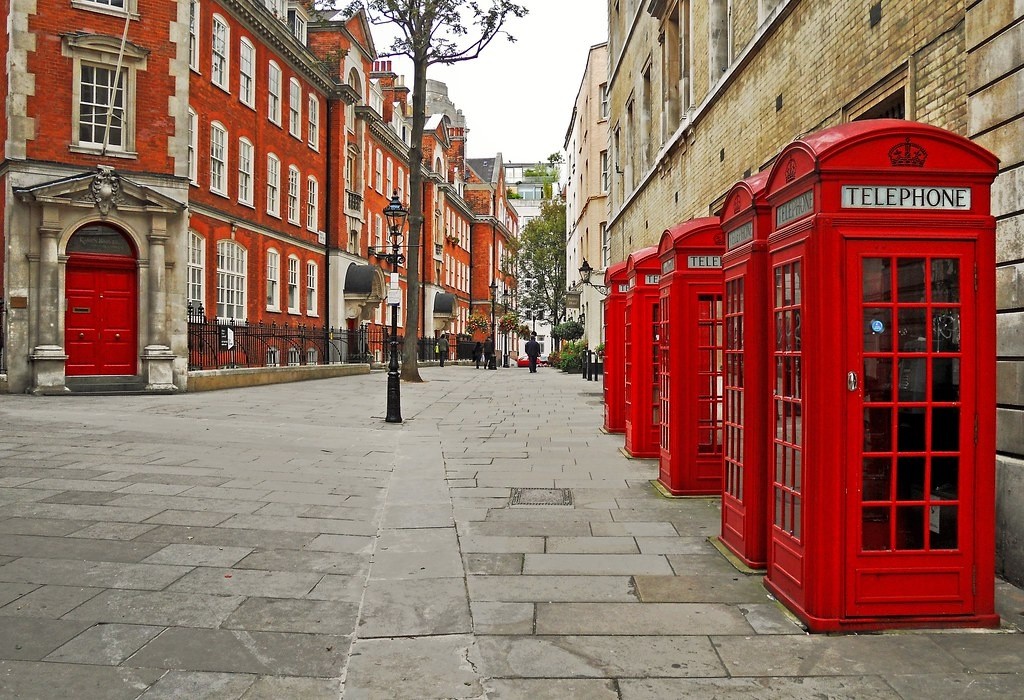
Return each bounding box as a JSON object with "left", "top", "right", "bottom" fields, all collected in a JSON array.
[{"left": 436, "top": 342, "right": 439, "bottom": 353}]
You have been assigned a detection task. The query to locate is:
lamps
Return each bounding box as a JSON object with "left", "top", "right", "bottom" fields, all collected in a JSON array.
[{"left": 578, "top": 257, "right": 608, "bottom": 296}]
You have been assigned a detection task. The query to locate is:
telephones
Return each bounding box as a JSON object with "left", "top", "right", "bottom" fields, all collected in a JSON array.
[{"left": 899, "top": 339, "right": 927, "bottom": 393}]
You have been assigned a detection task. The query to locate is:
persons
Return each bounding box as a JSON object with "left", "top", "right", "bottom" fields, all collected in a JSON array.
[
  {"left": 483, "top": 336, "right": 495, "bottom": 370},
  {"left": 474, "top": 341, "right": 483, "bottom": 369},
  {"left": 525, "top": 335, "right": 541, "bottom": 373},
  {"left": 437, "top": 334, "right": 450, "bottom": 367}
]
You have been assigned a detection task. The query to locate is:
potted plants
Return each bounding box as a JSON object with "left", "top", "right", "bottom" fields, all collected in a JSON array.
[
  {"left": 562, "top": 352, "right": 571, "bottom": 372},
  {"left": 453, "top": 237, "right": 460, "bottom": 245},
  {"left": 565, "top": 352, "right": 583, "bottom": 374},
  {"left": 448, "top": 235, "right": 456, "bottom": 243}
]
